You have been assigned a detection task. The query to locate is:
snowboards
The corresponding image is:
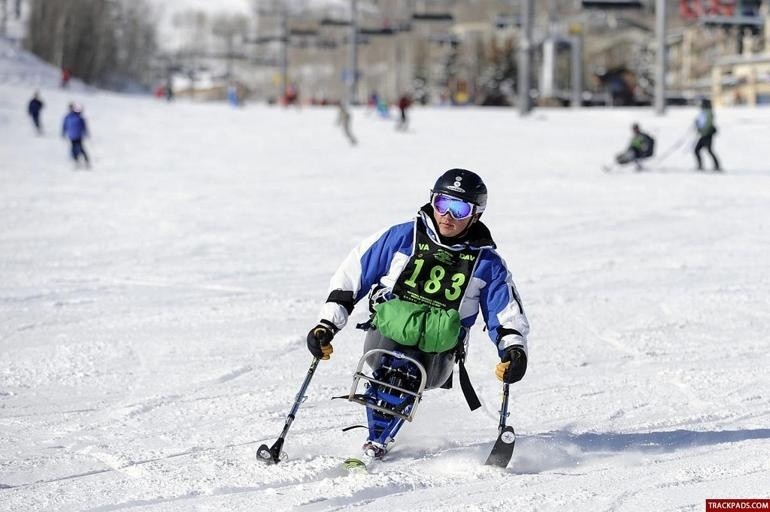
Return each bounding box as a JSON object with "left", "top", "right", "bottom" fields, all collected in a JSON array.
[{"left": 340, "top": 439, "right": 397, "bottom": 474}]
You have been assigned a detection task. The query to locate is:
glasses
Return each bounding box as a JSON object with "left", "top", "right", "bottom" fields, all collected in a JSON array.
[{"left": 430, "top": 193, "right": 486, "bottom": 221}]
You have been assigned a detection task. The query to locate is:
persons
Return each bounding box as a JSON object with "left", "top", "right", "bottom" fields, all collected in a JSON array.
[
  {"left": 334, "top": 99, "right": 359, "bottom": 146},
  {"left": 305, "top": 163, "right": 532, "bottom": 460},
  {"left": 692, "top": 98, "right": 723, "bottom": 171},
  {"left": 627, "top": 119, "right": 656, "bottom": 173},
  {"left": 397, "top": 91, "right": 415, "bottom": 122},
  {"left": 25, "top": 91, "right": 43, "bottom": 129},
  {"left": 60, "top": 101, "right": 88, "bottom": 162}
]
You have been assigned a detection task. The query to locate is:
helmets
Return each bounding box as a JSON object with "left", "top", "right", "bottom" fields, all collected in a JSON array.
[{"left": 431, "top": 169, "right": 487, "bottom": 206}]
